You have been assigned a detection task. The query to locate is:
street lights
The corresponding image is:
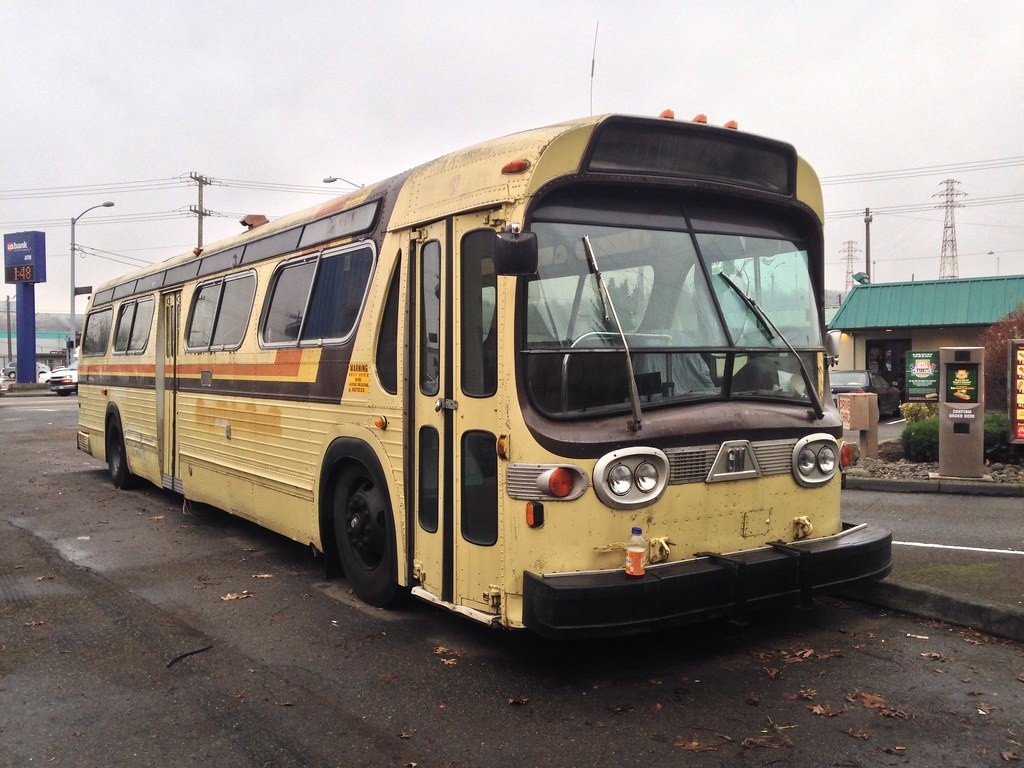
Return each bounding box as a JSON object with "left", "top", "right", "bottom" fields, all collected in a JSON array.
[
  {"left": 69, "top": 196, "right": 117, "bottom": 380},
  {"left": 321, "top": 174, "right": 362, "bottom": 190}
]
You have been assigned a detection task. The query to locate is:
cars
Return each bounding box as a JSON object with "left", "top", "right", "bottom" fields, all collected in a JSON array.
[
  {"left": 2, "top": 361, "right": 50, "bottom": 379},
  {"left": 827, "top": 365, "right": 902, "bottom": 418},
  {"left": 46, "top": 359, "right": 79, "bottom": 398}
]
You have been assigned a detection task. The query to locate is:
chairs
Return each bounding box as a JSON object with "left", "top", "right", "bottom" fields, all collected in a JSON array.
[{"left": 482, "top": 295, "right": 715, "bottom": 403}]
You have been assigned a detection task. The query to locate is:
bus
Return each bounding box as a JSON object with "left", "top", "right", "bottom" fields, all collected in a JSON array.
[{"left": 71, "top": 108, "right": 891, "bottom": 636}]
